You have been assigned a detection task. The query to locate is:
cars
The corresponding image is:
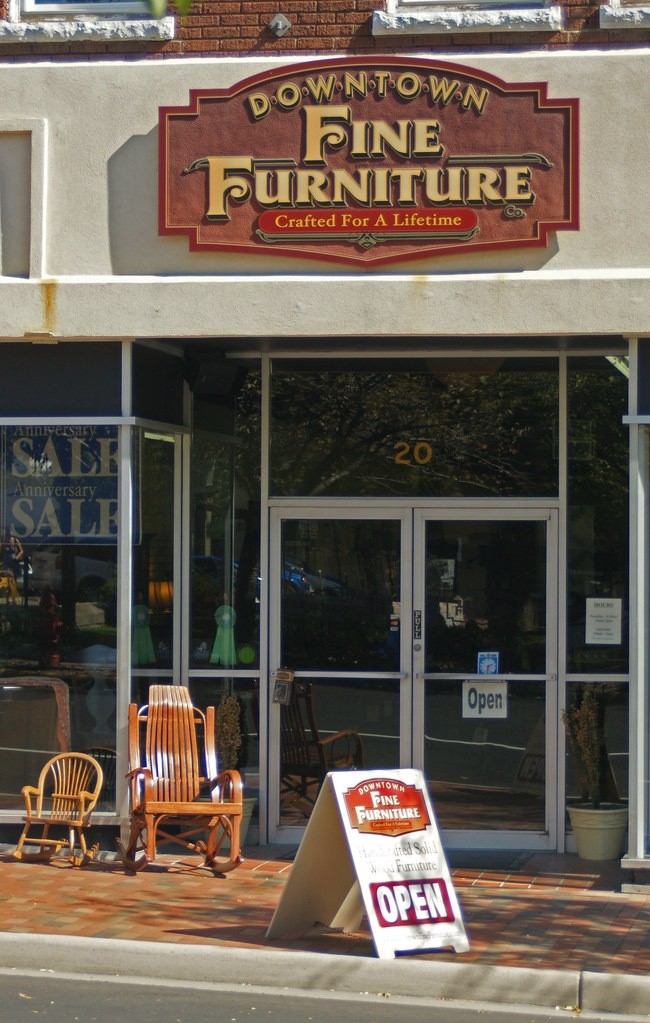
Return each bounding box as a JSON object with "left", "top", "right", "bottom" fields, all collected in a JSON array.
[{"left": 2, "top": 552, "right": 345, "bottom": 645}]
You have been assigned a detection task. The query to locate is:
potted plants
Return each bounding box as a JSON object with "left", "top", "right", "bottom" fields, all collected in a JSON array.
[{"left": 561, "top": 690, "right": 628, "bottom": 860}]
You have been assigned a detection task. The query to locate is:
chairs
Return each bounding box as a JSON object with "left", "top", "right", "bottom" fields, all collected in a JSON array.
[
  {"left": 10, "top": 751, "right": 104, "bottom": 866},
  {"left": 252, "top": 682, "right": 365, "bottom": 820},
  {"left": 113, "top": 684, "right": 244, "bottom": 874}
]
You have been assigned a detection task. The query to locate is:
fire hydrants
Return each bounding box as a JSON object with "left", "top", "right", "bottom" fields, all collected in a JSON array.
[{"left": 33, "top": 585, "right": 66, "bottom": 669}]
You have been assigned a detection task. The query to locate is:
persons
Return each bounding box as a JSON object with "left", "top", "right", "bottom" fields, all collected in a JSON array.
[{"left": 0, "top": 522, "right": 24, "bottom": 605}]
